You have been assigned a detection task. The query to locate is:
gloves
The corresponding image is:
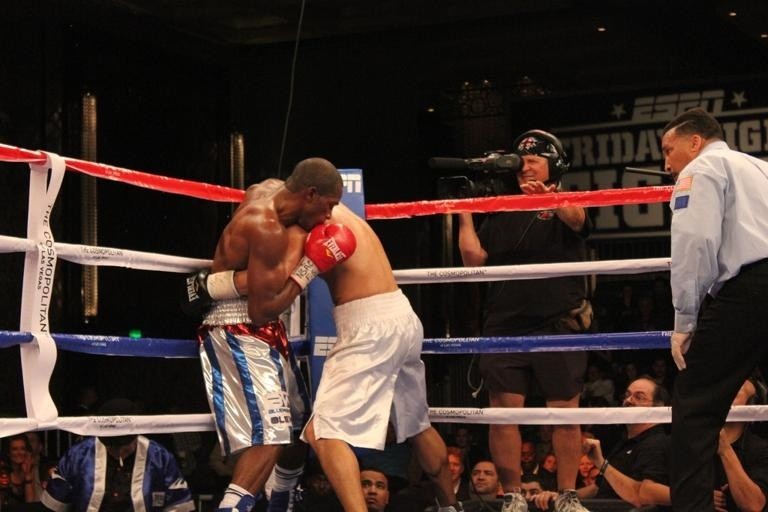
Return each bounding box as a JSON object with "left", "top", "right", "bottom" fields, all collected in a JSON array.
[
  {"left": 289, "top": 221, "right": 357, "bottom": 291},
  {"left": 177, "top": 269, "right": 241, "bottom": 314}
]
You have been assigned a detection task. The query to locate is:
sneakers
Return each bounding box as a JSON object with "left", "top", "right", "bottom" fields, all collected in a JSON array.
[
  {"left": 498, "top": 488, "right": 528, "bottom": 512},
  {"left": 554, "top": 489, "right": 591, "bottom": 512},
  {"left": 262, "top": 469, "right": 297, "bottom": 510}
]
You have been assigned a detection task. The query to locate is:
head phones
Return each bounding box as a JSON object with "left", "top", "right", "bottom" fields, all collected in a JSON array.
[{"left": 511, "top": 130, "right": 570, "bottom": 175}]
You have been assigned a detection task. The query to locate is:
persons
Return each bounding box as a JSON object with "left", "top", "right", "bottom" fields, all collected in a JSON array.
[
  {"left": 192, "top": 158, "right": 355, "bottom": 512},
  {"left": 661, "top": 107, "right": 768, "bottom": 512},
  {"left": 456, "top": 128, "right": 595, "bottom": 512},
  {"left": 181, "top": 198, "right": 459, "bottom": 512}
]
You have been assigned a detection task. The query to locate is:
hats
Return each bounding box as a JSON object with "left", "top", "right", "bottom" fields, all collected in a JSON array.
[{"left": 96, "top": 398, "right": 140, "bottom": 446}]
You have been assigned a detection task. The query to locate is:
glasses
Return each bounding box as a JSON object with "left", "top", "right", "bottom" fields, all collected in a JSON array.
[{"left": 617, "top": 392, "right": 652, "bottom": 404}]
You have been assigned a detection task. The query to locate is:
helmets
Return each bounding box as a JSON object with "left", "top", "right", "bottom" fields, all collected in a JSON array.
[{"left": 512, "top": 129, "right": 572, "bottom": 184}]
